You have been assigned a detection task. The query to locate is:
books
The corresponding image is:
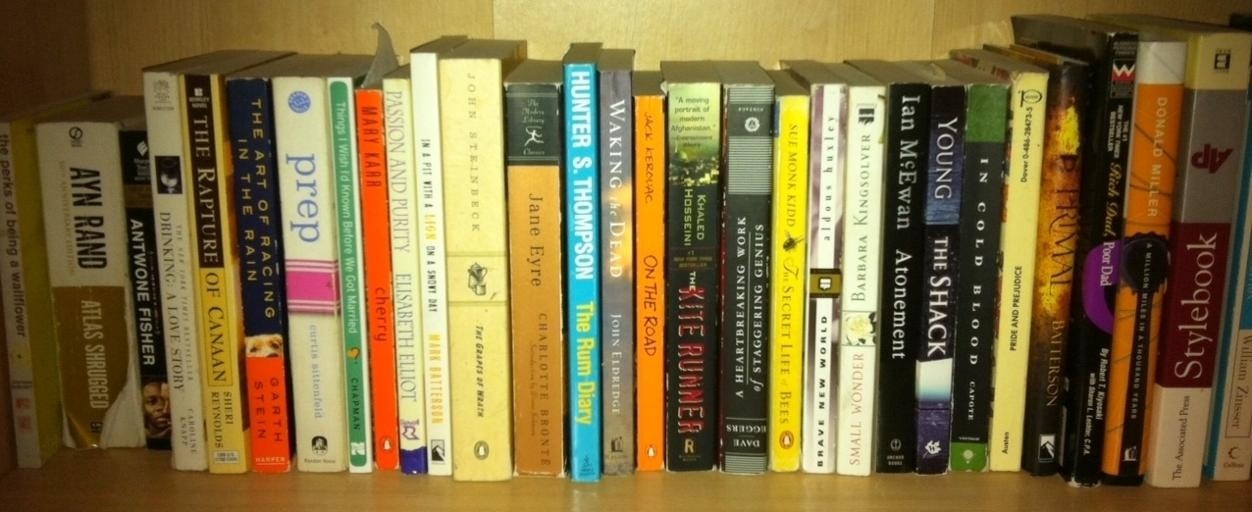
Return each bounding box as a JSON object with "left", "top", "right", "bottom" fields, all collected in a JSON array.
[{"left": 0, "top": 19, "right": 1249, "bottom": 486}]
